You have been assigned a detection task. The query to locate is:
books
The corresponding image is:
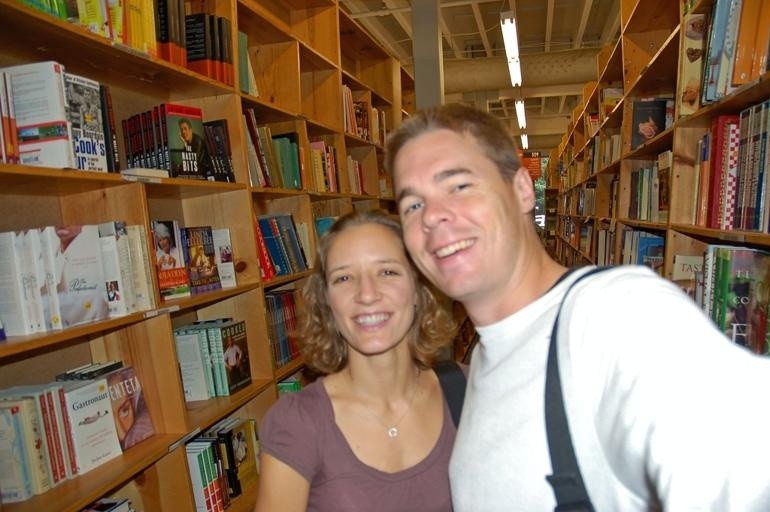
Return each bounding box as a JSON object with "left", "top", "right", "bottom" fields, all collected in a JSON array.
[
  {"left": 542, "top": 92, "right": 675, "bottom": 278},
  {"left": 265, "top": 290, "right": 304, "bottom": 370},
  {"left": 1, "top": 1, "right": 262, "bottom": 96},
  {"left": 173, "top": 318, "right": 253, "bottom": 401},
  {"left": 1, "top": 214, "right": 349, "bottom": 338},
  {"left": 0, "top": 361, "right": 160, "bottom": 512},
  {"left": 342, "top": 82, "right": 387, "bottom": 147},
  {"left": 277, "top": 378, "right": 302, "bottom": 396},
  {"left": 187, "top": 415, "right": 261, "bottom": 511},
  {"left": 2, "top": 61, "right": 367, "bottom": 195}
]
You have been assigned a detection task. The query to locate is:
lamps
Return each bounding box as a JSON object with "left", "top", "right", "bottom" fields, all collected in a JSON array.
[{"left": 498, "top": 11, "right": 530, "bottom": 151}]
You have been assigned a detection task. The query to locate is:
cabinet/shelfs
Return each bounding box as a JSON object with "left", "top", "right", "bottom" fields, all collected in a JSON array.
[
  {"left": 0, "top": 0, "right": 415, "bottom": 512},
  {"left": 545, "top": 1, "right": 770, "bottom": 357}
]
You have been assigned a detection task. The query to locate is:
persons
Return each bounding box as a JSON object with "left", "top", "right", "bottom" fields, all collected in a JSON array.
[
  {"left": 682, "top": 0, "right": 770, "bottom": 359},
  {"left": 378, "top": 104, "right": 770, "bottom": 511},
  {"left": 248, "top": 206, "right": 474, "bottom": 512}
]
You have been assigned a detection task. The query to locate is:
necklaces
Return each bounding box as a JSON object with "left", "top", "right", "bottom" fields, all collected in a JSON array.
[{"left": 348, "top": 368, "right": 419, "bottom": 437}]
